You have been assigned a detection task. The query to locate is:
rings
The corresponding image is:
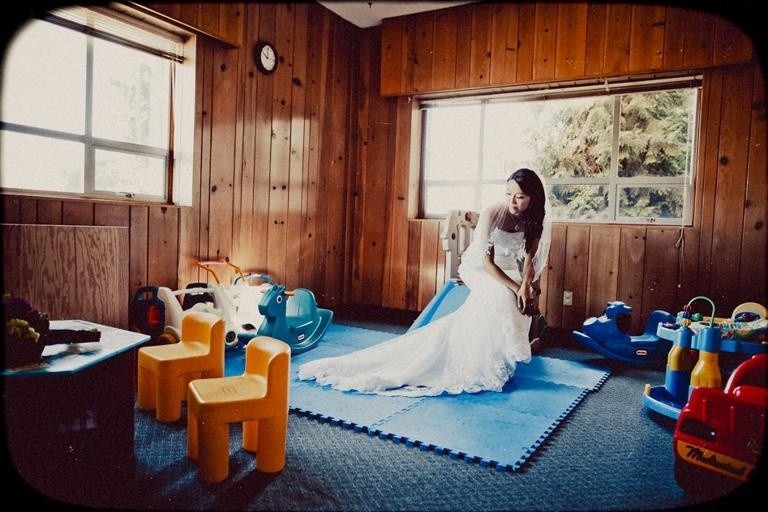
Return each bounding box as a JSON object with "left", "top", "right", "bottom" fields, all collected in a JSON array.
[{"left": 529, "top": 302, "right": 535, "bottom": 308}]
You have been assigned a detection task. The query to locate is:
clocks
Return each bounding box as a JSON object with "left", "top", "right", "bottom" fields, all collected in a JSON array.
[{"left": 254, "top": 43, "right": 279, "bottom": 76}]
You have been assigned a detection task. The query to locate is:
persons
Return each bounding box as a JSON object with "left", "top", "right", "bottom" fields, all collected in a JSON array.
[{"left": 456, "top": 168, "right": 552, "bottom": 396}]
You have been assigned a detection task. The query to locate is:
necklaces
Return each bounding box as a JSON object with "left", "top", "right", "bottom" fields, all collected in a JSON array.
[{"left": 508, "top": 214, "right": 525, "bottom": 232}]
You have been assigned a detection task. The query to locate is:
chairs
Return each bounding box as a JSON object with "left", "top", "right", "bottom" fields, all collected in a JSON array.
[
  {"left": 186, "top": 335, "right": 289, "bottom": 483},
  {"left": 137, "top": 311, "right": 226, "bottom": 423}
]
